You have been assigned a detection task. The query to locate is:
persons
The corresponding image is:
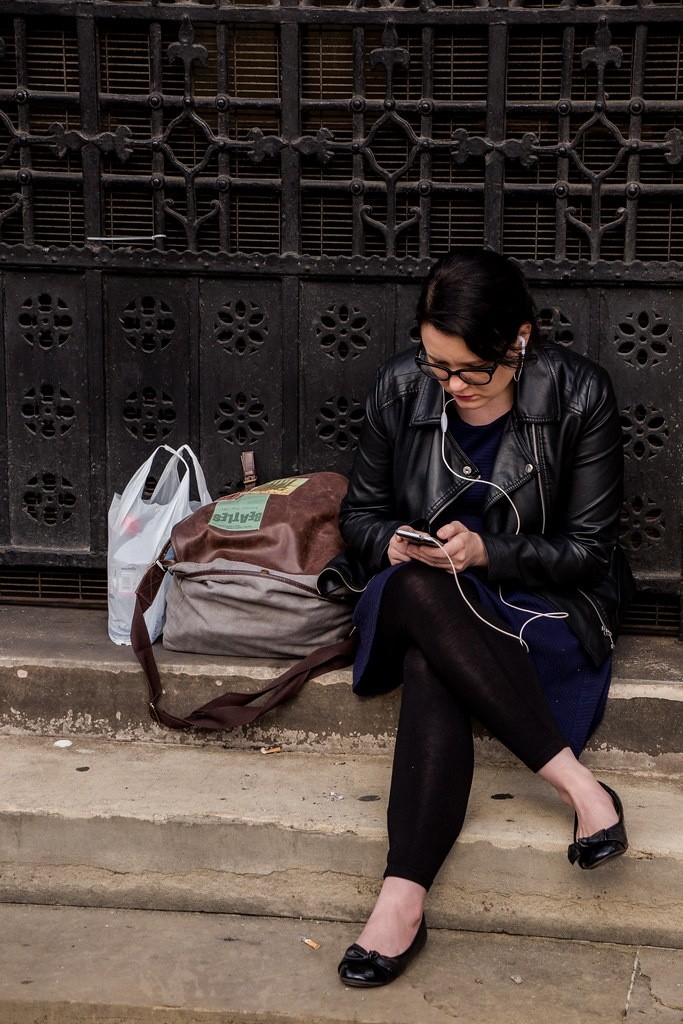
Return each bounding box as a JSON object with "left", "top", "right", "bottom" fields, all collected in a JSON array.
[{"left": 338, "top": 247, "right": 635, "bottom": 989}]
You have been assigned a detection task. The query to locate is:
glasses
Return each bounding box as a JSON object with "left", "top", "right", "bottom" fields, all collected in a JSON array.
[{"left": 415, "top": 337, "right": 515, "bottom": 386}]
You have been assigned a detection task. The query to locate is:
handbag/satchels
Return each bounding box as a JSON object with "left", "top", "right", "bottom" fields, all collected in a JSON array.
[
  {"left": 107, "top": 444, "right": 214, "bottom": 647},
  {"left": 169, "top": 450, "right": 349, "bottom": 576},
  {"left": 162, "top": 556, "right": 357, "bottom": 661}
]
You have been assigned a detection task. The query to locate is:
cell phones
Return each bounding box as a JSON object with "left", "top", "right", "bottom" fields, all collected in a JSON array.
[{"left": 394, "top": 530, "right": 444, "bottom": 549}]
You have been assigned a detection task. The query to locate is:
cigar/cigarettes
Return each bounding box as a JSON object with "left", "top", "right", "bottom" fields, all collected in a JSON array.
[
  {"left": 260, "top": 747, "right": 281, "bottom": 754},
  {"left": 304, "top": 939, "right": 320, "bottom": 949}
]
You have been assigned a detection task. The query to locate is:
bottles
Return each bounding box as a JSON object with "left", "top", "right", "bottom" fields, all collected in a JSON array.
[{"left": 110, "top": 519, "right": 148, "bottom": 646}]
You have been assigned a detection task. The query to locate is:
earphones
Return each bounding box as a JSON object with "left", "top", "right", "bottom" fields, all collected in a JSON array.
[{"left": 518, "top": 336, "right": 525, "bottom": 356}]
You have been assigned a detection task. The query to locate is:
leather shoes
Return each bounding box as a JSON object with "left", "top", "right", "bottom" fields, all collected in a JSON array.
[
  {"left": 567, "top": 778, "right": 629, "bottom": 870},
  {"left": 337, "top": 910, "right": 428, "bottom": 989}
]
